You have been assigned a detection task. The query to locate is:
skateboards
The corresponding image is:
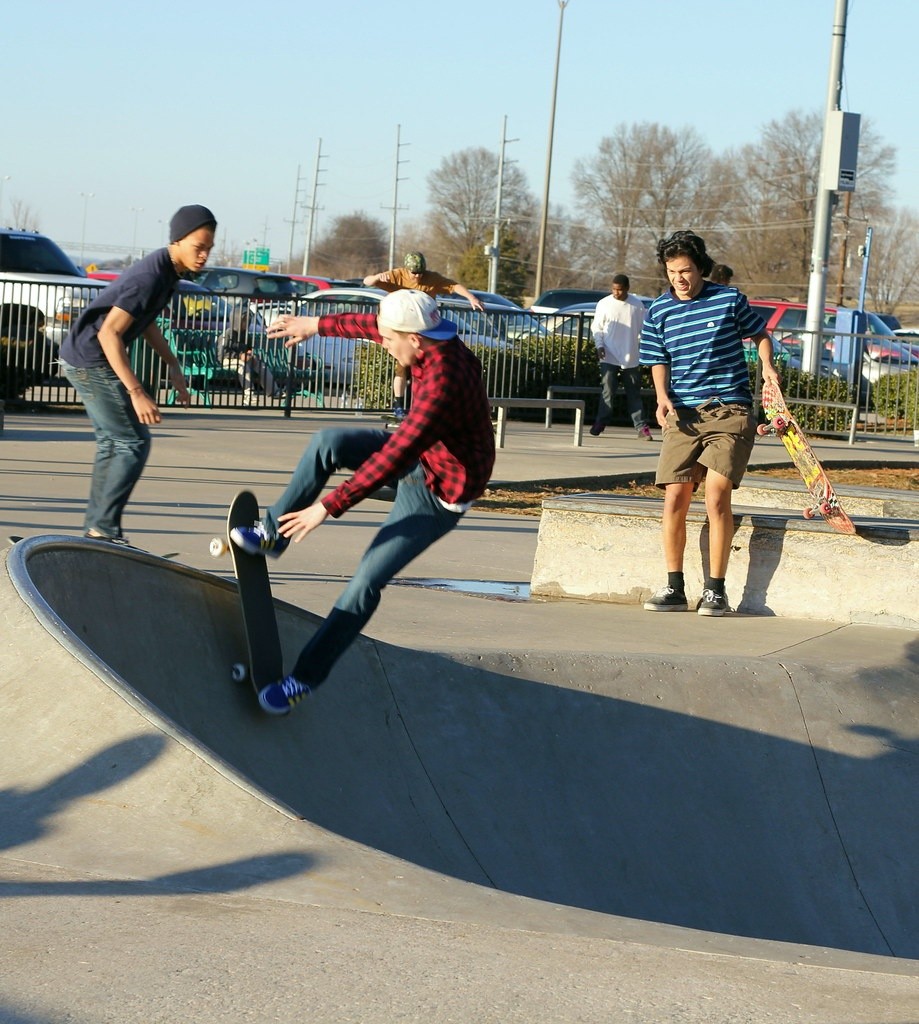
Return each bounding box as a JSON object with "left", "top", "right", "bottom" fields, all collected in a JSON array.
[
  {"left": 207, "top": 487, "right": 292, "bottom": 719},
  {"left": 379, "top": 414, "right": 405, "bottom": 428},
  {"left": 755, "top": 379, "right": 858, "bottom": 537},
  {"left": 7, "top": 534, "right": 181, "bottom": 560}
]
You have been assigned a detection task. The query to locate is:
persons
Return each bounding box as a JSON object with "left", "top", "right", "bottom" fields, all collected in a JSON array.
[
  {"left": 231, "top": 288, "right": 496, "bottom": 714},
  {"left": 216, "top": 306, "right": 277, "bottom": 395},
  {"left": 711, "top": 264, "right": 734, "bottom": 287},
  {"left": 59, "top": 204, "right": 217, "bottom": 546},
  {"left": 589, "top": 275, "right": 653, "bottom": 441},
  {"left": 363, "top": 251, "right": 484, "bottom": 418},
  {"left": 638, "top": 230, "right": 783, "bottom": 616}
]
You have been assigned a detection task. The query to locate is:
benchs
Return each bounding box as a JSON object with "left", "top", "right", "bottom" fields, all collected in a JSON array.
[{"left": 163, "top": 328, "right": 327, "bottom": 413}]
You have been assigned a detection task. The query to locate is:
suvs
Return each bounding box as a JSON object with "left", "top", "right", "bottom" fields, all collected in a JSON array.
[{"left": 0, "top": 227, "right": 125, "bottom": 348}]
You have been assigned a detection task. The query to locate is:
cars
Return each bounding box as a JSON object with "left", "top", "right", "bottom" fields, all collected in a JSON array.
[
  {"left": 744, "top": 300, "right": 919, "bottom": 385},
  {"left": 444, "top": 289, "right": 556, "bottom": 345},
  {"left": 86, "top": 268, "right": 307, "bottom": 389},
  {"left": 187, "top": 263, "right": 373, "bottom": 307},
  {"left": 252, "top": 287, "right": 516, "bottom": 389},
  {"left": 543, "top": 300, "right": 602, "bottom": 345},
  {"left": 1, "top": 302, "right": 62, "bottom": 401},
  {"left": 529, "top": 287, "right": 655, "bottom": 332}
]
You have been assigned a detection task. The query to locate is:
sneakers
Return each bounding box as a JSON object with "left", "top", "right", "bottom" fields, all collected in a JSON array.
[
  {"left": 230, "top": 515, "right": 291, "bottom": 559},
  {"left": 643, "top": 585, "right": 687, "bottom": 612},
  {"left": 696, "top": 588, "right": 729, "bottom": 617},
  {"left": 259, "top": 672, "right": 313, "bottom": 717}
]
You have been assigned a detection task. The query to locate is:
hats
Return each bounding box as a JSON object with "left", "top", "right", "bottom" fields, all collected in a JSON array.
[
  {"left": 170, "top": 206, "right": 218, "bottom": 245},
  {"left": 377, "top": 289, "right": 457, "bottom": 341},
  {"left": 407, "top": 253, "right": 426, "bottom": 274}
]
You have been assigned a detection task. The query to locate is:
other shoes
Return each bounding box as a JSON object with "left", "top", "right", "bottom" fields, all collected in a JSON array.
[
  {"left": 85, "top": 529, "right": 131, "bottom": 546},
  {"left": 590, "top": 423, "right": 606, "bottom": 435},
  {"left": 243, "top": 394, "right": 257, "bottom": 402},
  {"left": 637, "top": 427, "right": 653, "bottom": 440},
  {"left": 273, "top": 390, "right": 287, "bottom": 400}
]
